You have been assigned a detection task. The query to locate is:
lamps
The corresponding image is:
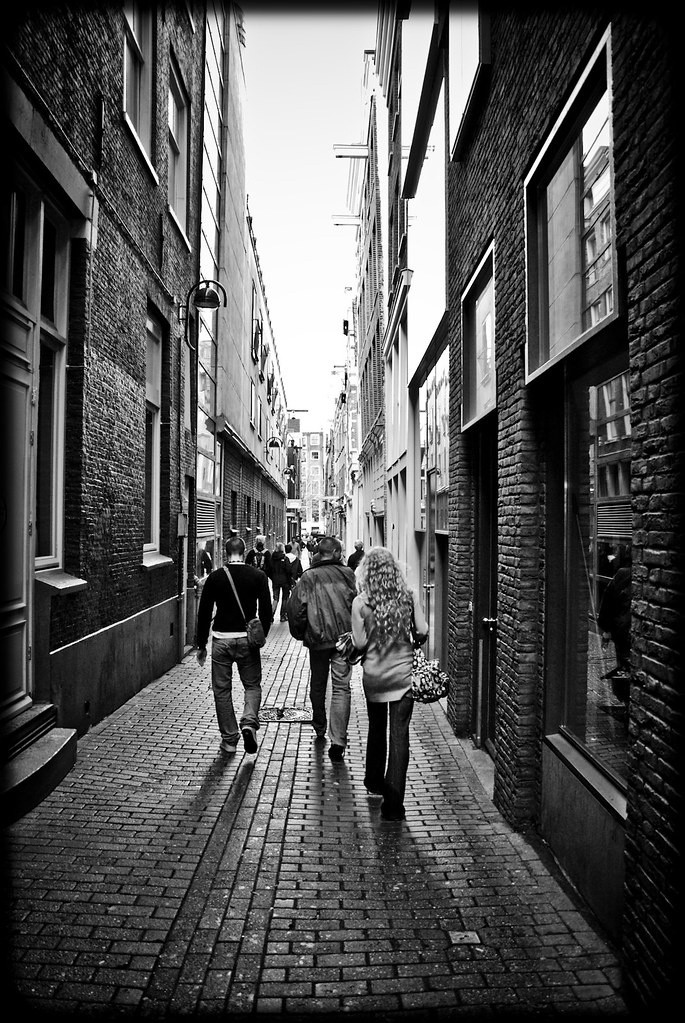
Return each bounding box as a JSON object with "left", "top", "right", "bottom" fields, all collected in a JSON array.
[
  {"left": 265, "top": 436, "right": 283, "bottom": 465},
  {"left": 177, "top": 280, "right": 227, "bottom": 352},
  {"left": 282, "top": 466, "right": 292, "bottom": 486}
]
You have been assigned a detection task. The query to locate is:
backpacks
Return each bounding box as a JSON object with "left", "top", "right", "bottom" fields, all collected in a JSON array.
[{"left": 251, "top": 547, "right": 268, "bottom": 572}]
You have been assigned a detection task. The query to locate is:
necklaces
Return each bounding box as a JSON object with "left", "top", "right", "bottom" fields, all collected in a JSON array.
[{"left": 229, "top": 560, "right": 244, "bottom": 562}]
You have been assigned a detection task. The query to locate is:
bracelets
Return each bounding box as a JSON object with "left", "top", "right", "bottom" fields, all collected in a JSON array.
[{"left": 198, "top": 646, "right": 205, "bottom": 650}]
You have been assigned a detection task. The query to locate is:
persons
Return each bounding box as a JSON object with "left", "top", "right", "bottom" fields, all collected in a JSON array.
[
  {"left": 285, "top": 544, "right": 303, "bottom": 586},
  {"left": 288, "top": 537, "right": 358, "bottom": 756},
  {"left": 245, "top": 534, "right": 272, "bottom": 581},
  {"left": 194, "top": 537, "right": 272, "bottom": 754},
  {"left": 288, "top": 531, "right": 345, "bottom": 567},
  {"left": 598, "top": 542, "right": 632, "bottom": 706},
  {"left": 349, "top": 548, "right": 430, "bottom": 818},
  {"left": 270, "top": 542, "right": 291, "bottom": 622},
  {"left": 199, "top": 549, "right": 213, "bottom": 577},
  {"left": 347, "top": 540, "right": 365, "bottom": 571}
]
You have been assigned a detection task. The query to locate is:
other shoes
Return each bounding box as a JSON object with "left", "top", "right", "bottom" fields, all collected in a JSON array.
[
  {"left": 312, "top": 718, "right": 327, "bottom": 737},
  {"left": 242, "top": 726, "right": 258, "bottom": 754},
  {"left": 381, "top": 802, "right": 406, "bottom": 820},
  {"left": 327, "top": 743, "right": 344, "bottom": 758},
  {"left": 220, "top": 740, "right": 237, "bottom": 752},
  {"left": 280, "top": 616, "right": 288, "bottom": 622},
  {"left": 363, "top": 773, "right": 385, "bottom": 795}
]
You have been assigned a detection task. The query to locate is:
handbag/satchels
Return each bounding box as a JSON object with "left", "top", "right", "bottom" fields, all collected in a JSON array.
[
  {"left": 411, "top": 648, "right": 450, "bottom": 703},
  {"left": 334, "top": 629, "right": 369, "bottom": 665},
  {"left": 247, "top": 618, "right": 266, "bottom": 648}
]
